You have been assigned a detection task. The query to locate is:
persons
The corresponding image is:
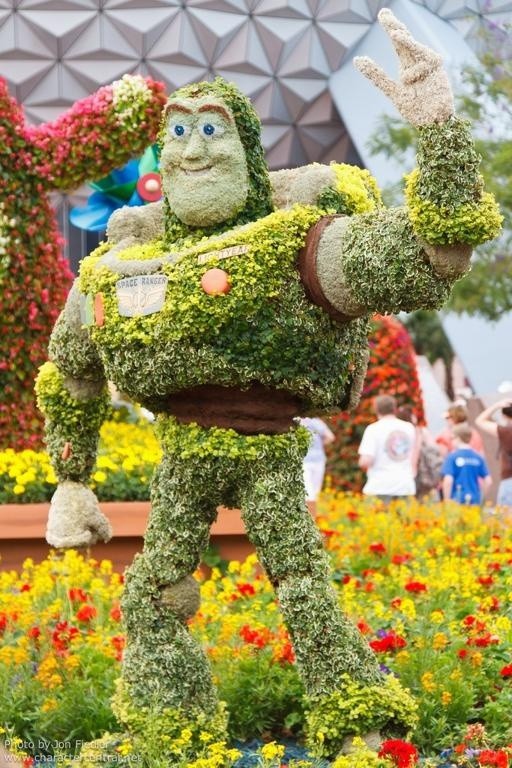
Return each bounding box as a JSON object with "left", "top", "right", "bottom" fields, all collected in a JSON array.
[
  {"left": 397, "top": 403, "right": 440, "bottom": 498},
  {"left": 298, "top": 416, "right": 336, "bottom": 502},
  {"left": 473, "top": 399, "right": 512, "bottom": 507},
  {"left": 437, "top": 401, "right": 483, "bottom": 499},
  {"left": 356, "top": 394, "right": 419, "bottom": 501},
  {"left": 442, "top": 427, "right": 491, "bottom": 505}
]
